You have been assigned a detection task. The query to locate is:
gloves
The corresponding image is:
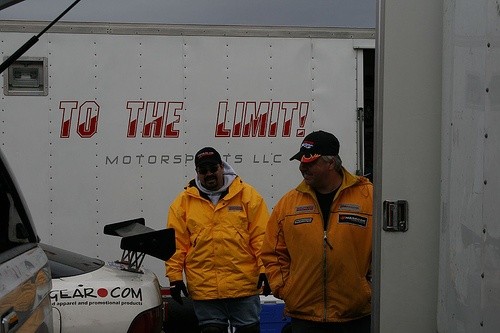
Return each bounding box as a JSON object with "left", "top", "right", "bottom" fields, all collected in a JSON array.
[
  {"left": 257, "top": 273, "right": 271, "bottom": 296},
  {"left": 170, "top": 280, "right": 189, "bottom": 305}
]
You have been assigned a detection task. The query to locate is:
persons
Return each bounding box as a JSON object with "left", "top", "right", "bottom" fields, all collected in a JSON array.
[
  {"left": 259, "top": 129, "right": 374, "bottom": 333},
  {"left": 164, "top": 147, "right": 271, "bottom": 333}
]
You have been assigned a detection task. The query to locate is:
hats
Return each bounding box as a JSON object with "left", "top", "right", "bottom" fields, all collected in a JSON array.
[
  {"left": 289, "top": 131, "right": 340, "bottom": 163},
  {"left": 195, "top": 147, "right": 222, "bottom": 166}
]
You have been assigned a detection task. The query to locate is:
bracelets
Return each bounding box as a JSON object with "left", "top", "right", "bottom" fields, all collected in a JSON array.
[{"left": 366, "top": 275, "right": 373, "bottom": 282}]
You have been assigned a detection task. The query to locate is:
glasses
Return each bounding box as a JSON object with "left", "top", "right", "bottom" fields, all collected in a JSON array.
[{"left": 196, "top": 165, "right": 221, "bottom": 174}]
